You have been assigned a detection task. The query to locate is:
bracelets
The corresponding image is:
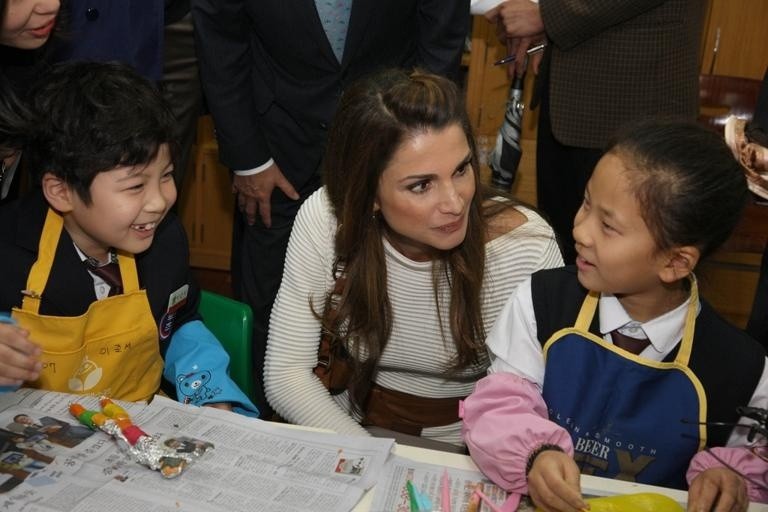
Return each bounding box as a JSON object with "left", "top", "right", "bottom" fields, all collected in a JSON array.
[{"left": 526, "top": 444, "right": 565, "bottom": 483}]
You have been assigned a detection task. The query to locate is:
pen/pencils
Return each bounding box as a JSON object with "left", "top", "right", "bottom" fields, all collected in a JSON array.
[{"left": 494, "top": 45, "right": 544, "bottom": 66}]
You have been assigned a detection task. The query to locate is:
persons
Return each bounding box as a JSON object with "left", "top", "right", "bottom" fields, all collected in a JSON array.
[
  {"left": 54, "top": 2, "right": 164, "bottom": 89},
  {"left": 483, "top": 2, "right": 708, "bottom": 266},
  {"left": 10, "top": 414, "right": 95, "bottom": 448},
  {"left": 459, "top": 115, "right": 766, "bottom": 511},
  {"left": 1, "top": 56, "right": 259, "bottom": 419},
  {"left": 164, "top": 439, "right": 208, "bottom": 461},
  {"left": 263, "top": 68, "right": 565, "bottom": 456},
  {"left": 191, "top": 1, "right": 474, "bottom": 422},
  {"left": 2, "top": 2, "right": 73, "bottom": 204}
]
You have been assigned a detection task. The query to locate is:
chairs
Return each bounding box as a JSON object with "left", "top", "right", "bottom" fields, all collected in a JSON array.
[{"left": 173, "top": 290, "right": 254, "bottom": 414}]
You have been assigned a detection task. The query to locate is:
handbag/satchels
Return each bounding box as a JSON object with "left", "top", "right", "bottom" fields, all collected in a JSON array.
[{"left": 314, "top": 354, "right": 355, "bottom": 396}]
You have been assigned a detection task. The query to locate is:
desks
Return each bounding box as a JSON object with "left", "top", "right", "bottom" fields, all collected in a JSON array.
[{"left": 1, "top": 384, "right": 767, "bottom": 511}]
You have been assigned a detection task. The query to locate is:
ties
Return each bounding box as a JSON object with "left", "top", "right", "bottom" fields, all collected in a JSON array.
[
  {"left": 611, "top": 329, "right": 650, "bottom": 356},
  {"left": 84, "top": 261, "right": 122, "bottom": 297}
]
